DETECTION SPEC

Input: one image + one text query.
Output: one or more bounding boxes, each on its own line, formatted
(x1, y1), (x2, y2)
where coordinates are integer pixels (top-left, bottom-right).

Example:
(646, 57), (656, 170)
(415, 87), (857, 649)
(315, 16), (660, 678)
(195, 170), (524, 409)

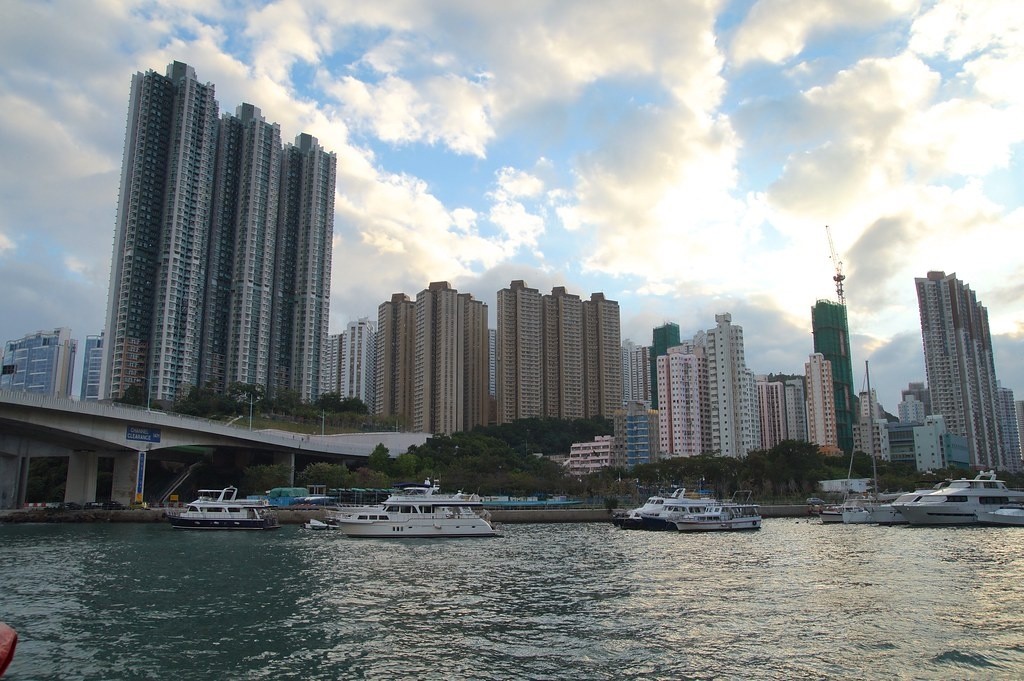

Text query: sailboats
(818, 360), (910, 525)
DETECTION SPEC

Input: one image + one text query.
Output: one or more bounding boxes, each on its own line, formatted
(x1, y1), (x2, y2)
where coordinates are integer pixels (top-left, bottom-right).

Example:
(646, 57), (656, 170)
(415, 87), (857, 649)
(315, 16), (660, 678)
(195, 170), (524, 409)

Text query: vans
(806, 498), (825, 506)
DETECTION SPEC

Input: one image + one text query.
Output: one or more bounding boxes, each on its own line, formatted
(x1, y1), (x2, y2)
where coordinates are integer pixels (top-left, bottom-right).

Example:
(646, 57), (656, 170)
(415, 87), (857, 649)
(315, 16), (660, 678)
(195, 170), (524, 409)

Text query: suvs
(61, 501), (148, 509)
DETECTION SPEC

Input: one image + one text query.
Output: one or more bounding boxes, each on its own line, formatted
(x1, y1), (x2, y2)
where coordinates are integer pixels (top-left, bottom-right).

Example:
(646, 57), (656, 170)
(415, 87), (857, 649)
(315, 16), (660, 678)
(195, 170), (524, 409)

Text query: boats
(674, 501), (761, 530)
(638, 496), (716, 529)
(300, 517), (340, 530)
(841, 491), (910, 523)
(976, 501), (1024, 524)
(163, 485), (281, 530)
(613, 488), (738, 530)
(890, 470), (1024, 526)
(338, 482), (503, 537)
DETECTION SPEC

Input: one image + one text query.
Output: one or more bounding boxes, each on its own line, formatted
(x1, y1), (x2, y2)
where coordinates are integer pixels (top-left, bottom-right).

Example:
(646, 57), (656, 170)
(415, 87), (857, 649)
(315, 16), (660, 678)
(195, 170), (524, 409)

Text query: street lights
(317, 409), (325, 435)
(244, 394), (252, 430)
(142, 376), (151, 412)
